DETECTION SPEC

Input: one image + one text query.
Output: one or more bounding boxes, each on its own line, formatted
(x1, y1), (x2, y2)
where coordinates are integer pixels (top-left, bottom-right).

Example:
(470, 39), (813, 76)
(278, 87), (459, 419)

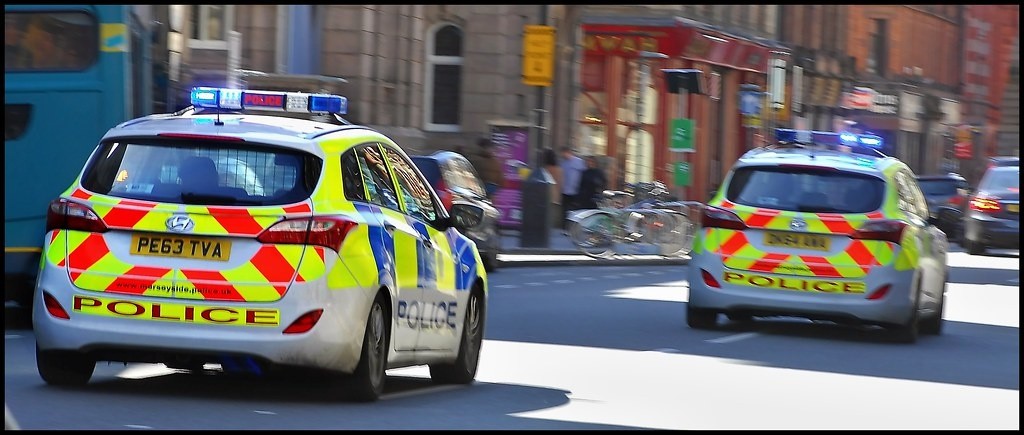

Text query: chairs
(151, 156), (248, 199)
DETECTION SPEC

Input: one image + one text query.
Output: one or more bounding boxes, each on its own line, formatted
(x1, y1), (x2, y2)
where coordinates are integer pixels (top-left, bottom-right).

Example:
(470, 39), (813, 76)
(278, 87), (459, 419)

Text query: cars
(685, 127), (949, 343)
(963, 156), (1019, 249)
(914, 173), (970, 238)
(403, 148), (501, 274)
(31, 88), (487, 404)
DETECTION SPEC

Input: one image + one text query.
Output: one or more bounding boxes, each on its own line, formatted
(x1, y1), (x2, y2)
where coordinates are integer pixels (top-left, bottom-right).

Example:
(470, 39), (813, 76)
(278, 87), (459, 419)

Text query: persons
(469, 137), (608, 249)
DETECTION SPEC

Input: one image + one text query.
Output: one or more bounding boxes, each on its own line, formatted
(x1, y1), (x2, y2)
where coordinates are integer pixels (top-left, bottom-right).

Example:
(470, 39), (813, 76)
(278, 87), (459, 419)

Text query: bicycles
(564, 181), (702, 258)
